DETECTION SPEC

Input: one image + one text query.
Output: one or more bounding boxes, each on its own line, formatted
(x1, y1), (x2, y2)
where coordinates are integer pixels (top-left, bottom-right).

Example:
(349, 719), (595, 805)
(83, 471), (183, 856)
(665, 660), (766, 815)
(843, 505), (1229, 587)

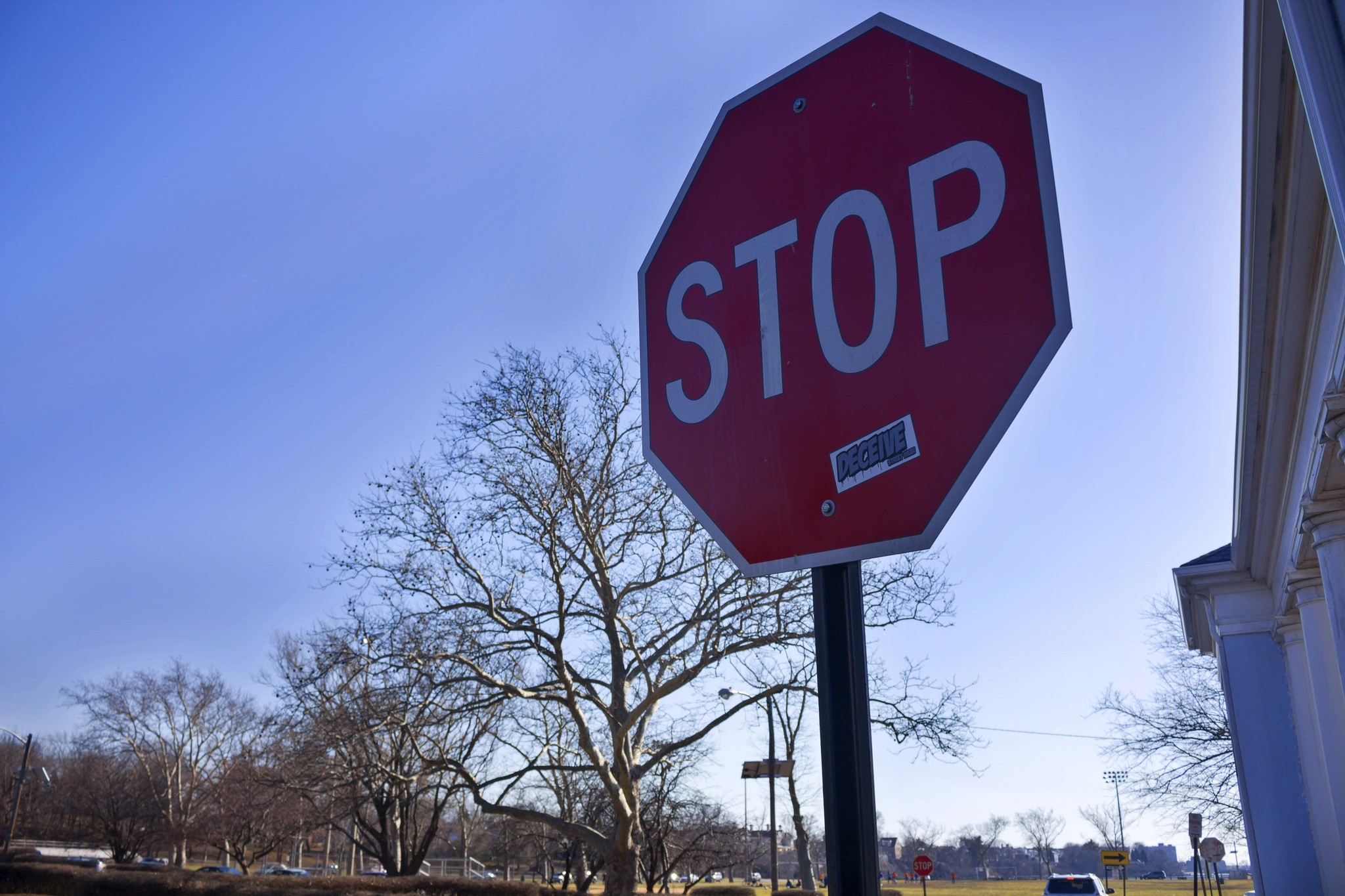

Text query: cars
(141, 855), (763, 884)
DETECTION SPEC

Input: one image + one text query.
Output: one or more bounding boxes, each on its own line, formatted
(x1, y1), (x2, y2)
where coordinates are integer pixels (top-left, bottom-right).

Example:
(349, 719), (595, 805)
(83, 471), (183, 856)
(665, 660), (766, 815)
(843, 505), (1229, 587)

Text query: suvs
(1141, 870), (1167, 880)
(1041, 873), (1115, 896)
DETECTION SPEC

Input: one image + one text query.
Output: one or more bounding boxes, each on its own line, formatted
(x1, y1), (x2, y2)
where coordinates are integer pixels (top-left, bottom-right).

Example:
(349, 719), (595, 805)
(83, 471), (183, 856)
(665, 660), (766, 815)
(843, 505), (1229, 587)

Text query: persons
(785, 867), (957, 891)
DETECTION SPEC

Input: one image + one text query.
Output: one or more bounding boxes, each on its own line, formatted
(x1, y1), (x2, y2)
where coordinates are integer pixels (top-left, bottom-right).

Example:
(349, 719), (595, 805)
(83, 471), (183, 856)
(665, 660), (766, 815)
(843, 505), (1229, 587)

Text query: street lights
(719, 687), (780, 893)
(1103, 771), (1129, 880)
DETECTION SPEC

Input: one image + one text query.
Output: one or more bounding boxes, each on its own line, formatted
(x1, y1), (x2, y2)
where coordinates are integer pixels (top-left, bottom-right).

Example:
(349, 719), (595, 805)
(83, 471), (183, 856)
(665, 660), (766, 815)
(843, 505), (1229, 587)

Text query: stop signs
(1199, 837), (1226, 862)
(911, 854), (935, 878)
(636, 12), (1072, 579)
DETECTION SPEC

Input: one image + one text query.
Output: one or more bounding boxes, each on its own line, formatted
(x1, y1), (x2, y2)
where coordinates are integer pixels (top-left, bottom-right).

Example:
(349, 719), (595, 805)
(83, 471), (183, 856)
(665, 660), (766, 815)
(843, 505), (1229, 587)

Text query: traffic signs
(1188, 813), (1202, 837)
(1105, 865), (1125, 871)
